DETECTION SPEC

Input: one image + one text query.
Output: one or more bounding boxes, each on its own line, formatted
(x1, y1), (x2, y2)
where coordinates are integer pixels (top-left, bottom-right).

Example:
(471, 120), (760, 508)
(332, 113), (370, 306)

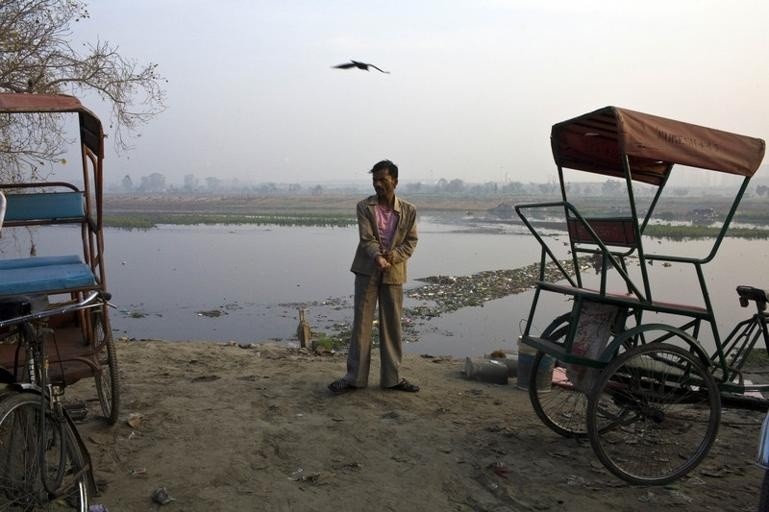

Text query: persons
(328, 160), (420, 392)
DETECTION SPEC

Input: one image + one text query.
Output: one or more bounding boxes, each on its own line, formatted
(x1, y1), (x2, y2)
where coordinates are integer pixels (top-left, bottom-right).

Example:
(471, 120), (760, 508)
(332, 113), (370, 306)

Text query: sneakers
(328, 378), (354, 393)
(382, 378), (420, 392)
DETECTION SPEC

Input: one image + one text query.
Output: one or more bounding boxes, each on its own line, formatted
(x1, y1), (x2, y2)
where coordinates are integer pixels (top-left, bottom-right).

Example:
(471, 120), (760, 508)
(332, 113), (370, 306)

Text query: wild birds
(330, 59), (391, 74)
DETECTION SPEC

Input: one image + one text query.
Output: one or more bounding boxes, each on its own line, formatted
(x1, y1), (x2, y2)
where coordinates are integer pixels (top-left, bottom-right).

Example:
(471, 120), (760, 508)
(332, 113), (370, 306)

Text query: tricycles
(0, 95), (121, 510)
(514, 105), (769, 487)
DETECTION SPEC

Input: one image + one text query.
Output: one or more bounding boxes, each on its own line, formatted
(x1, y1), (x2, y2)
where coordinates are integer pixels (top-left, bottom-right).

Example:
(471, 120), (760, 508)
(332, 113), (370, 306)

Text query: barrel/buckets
(517, 336), (554, 393)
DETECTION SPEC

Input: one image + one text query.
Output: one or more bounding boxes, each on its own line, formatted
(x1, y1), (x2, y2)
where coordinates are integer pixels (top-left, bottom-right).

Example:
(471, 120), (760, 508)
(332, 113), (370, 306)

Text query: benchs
(0, 181), (94, 300)
(537, 212), (711, 327)
